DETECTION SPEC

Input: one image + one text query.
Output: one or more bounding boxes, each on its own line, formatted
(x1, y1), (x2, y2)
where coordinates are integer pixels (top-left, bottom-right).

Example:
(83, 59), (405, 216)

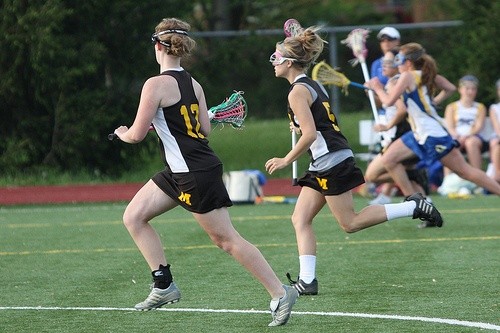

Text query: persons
(114, 18), (297, 326)
(364, 27), (500, 228)
(265, 29), (443, 295)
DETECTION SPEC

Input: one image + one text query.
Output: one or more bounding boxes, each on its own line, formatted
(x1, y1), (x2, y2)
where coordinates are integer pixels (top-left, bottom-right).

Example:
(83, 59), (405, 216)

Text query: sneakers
(286, 272), (319, 295)
(403, 192), (444, 226)
(267, 284), (299, 327)
(135, 281), (181, 312)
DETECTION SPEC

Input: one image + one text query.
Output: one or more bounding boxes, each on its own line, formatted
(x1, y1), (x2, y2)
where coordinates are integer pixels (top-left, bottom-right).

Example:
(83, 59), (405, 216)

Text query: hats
(377, 27), (401, 39)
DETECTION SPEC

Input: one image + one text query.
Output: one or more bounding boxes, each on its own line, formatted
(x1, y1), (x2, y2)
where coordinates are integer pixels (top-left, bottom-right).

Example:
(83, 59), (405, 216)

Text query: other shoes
(366, 194), (393, 205)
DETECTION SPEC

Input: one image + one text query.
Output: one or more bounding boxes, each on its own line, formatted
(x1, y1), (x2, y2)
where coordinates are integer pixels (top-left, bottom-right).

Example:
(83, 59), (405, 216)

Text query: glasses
(394, 54), (408, 65)
(151, 32), (170, 46)
(379, 37), (396, 41)
(269, 52), (303, 66)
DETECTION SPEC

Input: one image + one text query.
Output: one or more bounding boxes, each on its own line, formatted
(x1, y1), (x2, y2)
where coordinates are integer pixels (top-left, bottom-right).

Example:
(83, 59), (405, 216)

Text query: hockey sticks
(311, 61), (374, 90)
(345, 28), (380, 123)
(107, 90), (248, 141)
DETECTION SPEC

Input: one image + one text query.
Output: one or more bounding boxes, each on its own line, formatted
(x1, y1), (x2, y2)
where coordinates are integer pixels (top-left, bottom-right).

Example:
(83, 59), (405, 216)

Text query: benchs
(355, 116), (491, 161)
